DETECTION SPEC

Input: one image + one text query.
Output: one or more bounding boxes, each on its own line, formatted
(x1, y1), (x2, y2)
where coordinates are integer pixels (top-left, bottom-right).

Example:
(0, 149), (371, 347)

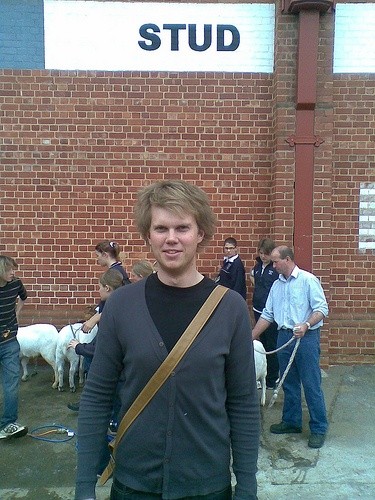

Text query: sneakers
(270, 422), (302, 434)
(0, 422), (28, 441)
(308, 434), (325, 448)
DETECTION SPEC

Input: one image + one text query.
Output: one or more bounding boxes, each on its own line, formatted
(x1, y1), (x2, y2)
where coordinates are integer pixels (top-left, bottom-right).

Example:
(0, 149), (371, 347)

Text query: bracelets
(306, 322), (311, 330)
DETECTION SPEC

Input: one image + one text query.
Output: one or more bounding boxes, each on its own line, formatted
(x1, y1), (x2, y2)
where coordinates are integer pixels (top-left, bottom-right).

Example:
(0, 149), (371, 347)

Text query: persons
(74, 180), (261, 500)
(133, 261), (156, 282)
(216, 237), (246, 301)
(251, 245), (329, 448)
(68, 269), (126, 435)
(251, 239), (279, 389)
(68, 241), (128, 411)
(0, 255), (28, 439)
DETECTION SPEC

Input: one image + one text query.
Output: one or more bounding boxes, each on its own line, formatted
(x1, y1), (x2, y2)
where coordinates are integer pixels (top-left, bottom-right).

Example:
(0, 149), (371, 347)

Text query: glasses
(224, 246), (236, 249)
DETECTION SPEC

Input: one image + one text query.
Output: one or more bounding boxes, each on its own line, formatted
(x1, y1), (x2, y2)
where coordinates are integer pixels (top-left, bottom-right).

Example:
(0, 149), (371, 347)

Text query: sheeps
(16, 324), (58, 389)
(253, 340), (267, 407)
(59, 318), (98, 391)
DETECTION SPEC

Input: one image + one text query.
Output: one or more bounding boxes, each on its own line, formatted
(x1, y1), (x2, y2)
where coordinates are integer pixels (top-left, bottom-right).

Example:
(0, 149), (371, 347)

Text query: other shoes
(275, 378), (279, 383)
(257, 381), (276, 389)
(68, 402), (80, 410)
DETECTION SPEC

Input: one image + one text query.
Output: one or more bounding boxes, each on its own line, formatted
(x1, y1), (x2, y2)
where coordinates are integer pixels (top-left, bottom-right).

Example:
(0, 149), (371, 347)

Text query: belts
(280, 328), (312, 334)
